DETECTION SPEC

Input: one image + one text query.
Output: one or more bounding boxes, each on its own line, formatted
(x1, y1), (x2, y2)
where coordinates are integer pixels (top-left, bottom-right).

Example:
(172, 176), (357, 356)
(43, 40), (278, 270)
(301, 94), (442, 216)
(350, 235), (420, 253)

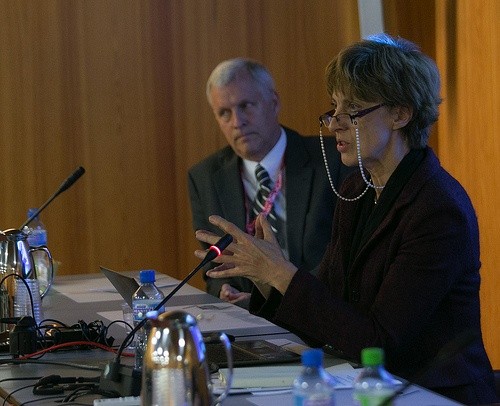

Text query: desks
(0, 270), (464, 406)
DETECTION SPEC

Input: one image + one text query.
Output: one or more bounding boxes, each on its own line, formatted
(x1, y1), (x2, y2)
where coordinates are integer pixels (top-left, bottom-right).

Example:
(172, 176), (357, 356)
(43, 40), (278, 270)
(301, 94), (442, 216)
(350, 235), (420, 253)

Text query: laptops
(98, 265), (301, 368)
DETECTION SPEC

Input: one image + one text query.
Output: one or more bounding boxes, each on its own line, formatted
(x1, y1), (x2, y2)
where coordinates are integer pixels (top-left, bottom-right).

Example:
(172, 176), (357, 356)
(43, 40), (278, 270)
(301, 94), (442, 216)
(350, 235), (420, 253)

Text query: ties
(252, 165), (279, 243)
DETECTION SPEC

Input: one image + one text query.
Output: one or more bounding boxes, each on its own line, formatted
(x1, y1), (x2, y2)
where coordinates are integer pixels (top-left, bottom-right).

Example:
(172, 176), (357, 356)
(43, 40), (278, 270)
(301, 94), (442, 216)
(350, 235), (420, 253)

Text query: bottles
(353, 348), (393, 406)
(23, 208), (48, 293)
(132, 270), (165, 368)
(292, 348), (337, 406)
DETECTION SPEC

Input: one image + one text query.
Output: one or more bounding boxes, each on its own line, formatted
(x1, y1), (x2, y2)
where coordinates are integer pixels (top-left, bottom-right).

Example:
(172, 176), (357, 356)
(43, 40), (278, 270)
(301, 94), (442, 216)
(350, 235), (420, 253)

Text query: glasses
(319, 100), (390, 128)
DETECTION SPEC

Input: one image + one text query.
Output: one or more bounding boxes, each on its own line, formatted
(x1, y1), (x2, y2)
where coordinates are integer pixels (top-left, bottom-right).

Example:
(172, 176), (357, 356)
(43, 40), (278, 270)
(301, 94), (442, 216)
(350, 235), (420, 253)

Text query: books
(218, 366), (306, 387)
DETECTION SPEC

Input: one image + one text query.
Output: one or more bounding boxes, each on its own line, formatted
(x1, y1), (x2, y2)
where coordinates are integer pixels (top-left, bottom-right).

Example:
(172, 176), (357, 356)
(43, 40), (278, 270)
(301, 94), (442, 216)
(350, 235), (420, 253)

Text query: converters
(50, 327), (101, 351)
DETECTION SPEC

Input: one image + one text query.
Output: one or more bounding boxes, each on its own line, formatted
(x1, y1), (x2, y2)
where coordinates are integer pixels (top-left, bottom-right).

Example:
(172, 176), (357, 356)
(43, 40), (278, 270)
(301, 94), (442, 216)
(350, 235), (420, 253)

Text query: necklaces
(373, 196), (377, 205)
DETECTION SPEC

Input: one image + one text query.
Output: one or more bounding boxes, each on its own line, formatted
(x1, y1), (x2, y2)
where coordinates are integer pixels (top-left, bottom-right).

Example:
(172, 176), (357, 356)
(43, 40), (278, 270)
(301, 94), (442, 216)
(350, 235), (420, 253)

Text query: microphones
(97, 233), (233, 396)
(18, 166), (85, 231)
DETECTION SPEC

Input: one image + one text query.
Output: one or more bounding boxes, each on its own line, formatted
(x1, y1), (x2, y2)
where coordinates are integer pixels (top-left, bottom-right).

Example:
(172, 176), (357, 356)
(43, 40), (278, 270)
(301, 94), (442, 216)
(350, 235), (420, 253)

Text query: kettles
(0, 228), (53, 343)
(141, 311), (235, 406)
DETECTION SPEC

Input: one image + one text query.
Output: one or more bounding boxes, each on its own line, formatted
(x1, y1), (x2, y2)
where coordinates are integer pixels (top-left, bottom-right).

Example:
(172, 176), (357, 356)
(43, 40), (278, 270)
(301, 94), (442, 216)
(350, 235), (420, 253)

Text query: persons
(187, 57), (357, 311)
(194, 35), (500, 406)
